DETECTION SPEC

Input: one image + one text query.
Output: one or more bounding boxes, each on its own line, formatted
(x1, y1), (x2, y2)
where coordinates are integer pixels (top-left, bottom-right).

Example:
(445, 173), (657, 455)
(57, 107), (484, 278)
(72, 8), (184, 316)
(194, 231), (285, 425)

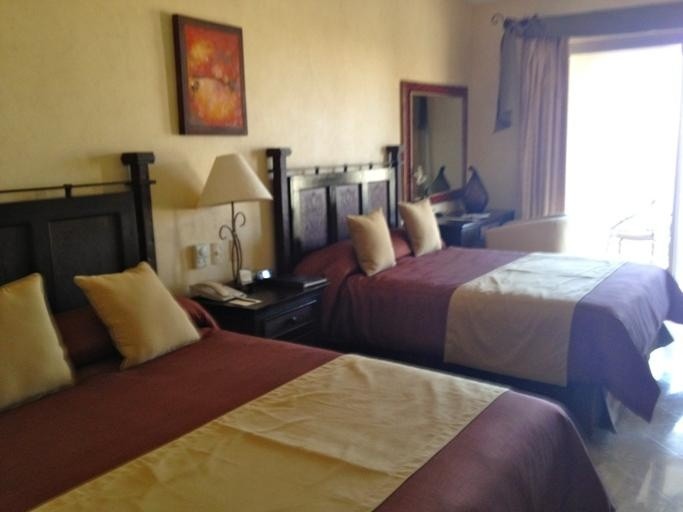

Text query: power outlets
(191, 243), (209, 269)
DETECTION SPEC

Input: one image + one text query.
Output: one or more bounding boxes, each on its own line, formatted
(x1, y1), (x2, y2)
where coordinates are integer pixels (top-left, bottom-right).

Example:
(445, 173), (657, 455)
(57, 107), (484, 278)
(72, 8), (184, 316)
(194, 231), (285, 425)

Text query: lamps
(191, 151), (273, 289)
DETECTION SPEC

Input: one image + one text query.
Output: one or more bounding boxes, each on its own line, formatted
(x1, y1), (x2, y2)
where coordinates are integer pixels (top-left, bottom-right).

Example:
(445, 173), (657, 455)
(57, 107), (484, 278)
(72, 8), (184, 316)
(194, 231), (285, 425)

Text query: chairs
(484, 210), (568, 253)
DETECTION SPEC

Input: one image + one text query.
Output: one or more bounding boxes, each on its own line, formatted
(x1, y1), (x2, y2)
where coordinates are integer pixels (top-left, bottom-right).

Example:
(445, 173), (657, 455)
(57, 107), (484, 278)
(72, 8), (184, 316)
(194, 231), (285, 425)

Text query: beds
(265, 145), (680, 434)
(0, 148), (615, 511)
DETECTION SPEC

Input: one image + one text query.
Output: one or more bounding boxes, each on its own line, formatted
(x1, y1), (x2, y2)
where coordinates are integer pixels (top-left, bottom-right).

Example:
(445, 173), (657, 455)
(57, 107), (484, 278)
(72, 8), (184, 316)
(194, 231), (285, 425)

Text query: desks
(434, 203), (513, 248)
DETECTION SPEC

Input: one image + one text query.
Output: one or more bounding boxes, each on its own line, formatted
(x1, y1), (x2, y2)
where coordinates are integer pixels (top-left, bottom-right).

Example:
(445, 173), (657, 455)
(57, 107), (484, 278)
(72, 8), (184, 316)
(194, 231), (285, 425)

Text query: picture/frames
(170, 9), (248, 136)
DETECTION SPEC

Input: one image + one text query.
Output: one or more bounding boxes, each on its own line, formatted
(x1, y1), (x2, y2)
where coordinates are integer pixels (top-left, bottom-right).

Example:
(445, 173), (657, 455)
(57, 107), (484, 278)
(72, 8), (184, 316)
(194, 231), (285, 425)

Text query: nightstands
(188, 272), (328, 348)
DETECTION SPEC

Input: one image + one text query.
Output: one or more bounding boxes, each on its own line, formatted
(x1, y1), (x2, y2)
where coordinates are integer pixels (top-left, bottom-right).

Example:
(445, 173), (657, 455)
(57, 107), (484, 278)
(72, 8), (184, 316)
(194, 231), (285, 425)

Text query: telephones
(189, 280), (244, 302)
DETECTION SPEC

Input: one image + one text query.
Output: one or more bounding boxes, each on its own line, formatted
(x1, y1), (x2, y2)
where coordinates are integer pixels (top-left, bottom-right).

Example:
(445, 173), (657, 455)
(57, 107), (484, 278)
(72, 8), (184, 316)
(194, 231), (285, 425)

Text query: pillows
(66, 252), (203, 374)
(338, 201), (398, 279)
(0, 273), (72, 415)
(398, 194), (445, 257)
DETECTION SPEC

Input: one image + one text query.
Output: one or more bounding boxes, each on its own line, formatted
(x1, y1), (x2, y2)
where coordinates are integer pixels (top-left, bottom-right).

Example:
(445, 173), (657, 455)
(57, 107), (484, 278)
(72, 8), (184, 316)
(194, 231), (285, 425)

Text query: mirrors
(399, 78), (467, 211)
